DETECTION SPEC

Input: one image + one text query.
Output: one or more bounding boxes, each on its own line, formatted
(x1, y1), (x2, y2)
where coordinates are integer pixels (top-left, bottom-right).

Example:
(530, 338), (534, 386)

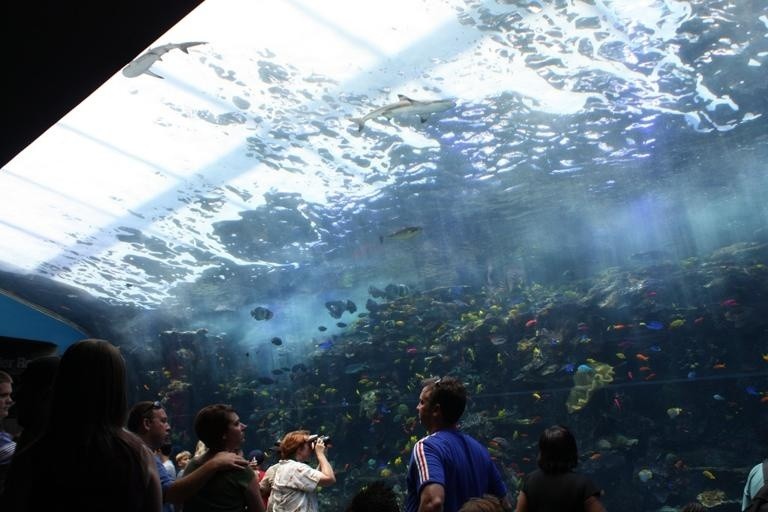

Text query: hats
(304, 434), (319, 443)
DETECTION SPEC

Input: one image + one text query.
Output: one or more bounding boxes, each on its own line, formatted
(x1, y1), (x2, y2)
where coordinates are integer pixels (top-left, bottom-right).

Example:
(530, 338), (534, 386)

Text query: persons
(0, 340), (162, 511)
(512, 421), (605, 512)
(404, 373), (508, 512)
(739, 458), (768, 512)
(125, 402), (336, 511)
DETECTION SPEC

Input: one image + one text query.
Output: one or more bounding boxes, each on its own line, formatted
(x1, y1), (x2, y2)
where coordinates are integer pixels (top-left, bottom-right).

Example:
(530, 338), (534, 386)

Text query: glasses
(144, 400), (163, 414)
(434, 377), (441, 389)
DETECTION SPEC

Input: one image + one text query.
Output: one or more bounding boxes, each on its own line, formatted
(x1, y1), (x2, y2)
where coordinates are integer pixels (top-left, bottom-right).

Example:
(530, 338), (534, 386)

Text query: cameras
(309, 435), (330, 451)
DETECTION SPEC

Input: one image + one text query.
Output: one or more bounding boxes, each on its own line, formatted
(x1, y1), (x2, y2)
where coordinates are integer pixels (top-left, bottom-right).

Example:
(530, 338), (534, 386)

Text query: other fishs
(135, 284), (768, 510)
